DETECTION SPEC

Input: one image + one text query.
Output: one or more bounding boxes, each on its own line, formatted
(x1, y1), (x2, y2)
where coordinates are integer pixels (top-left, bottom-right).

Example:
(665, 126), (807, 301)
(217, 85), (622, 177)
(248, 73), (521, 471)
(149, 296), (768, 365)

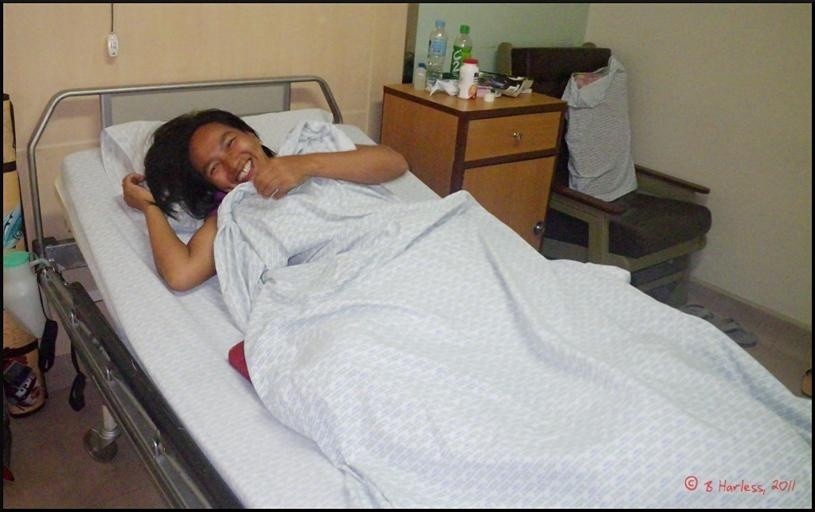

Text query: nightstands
(379, 81), (568, 255)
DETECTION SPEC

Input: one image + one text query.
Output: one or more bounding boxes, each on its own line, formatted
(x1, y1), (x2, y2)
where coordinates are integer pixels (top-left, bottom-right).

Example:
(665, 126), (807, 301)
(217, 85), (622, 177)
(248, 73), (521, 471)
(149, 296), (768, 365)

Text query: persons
(120, 108), (411, 292)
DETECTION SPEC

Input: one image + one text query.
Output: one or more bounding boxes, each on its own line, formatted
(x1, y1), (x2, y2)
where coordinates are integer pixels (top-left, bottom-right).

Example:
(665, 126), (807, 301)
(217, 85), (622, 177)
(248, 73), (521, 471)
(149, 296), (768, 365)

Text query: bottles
(413, 19), (477, 100)
(3, 251), (49, 341)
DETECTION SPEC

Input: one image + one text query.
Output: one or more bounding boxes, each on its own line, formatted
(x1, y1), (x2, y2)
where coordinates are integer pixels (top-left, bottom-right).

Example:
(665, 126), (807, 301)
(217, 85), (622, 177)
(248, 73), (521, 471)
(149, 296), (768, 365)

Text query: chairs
(494, 40), (712, 308)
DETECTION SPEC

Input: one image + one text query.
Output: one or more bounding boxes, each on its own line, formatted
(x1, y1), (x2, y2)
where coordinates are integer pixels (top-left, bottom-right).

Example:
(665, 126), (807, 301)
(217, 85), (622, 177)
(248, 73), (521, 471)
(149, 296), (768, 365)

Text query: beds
(59, 106), (813, 508)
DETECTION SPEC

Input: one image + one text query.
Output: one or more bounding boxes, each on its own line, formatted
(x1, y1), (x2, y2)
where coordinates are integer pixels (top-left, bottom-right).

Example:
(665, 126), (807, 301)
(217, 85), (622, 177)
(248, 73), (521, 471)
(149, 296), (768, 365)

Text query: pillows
(100, 108), (339, 236)
(561, 53), (640, 203)
